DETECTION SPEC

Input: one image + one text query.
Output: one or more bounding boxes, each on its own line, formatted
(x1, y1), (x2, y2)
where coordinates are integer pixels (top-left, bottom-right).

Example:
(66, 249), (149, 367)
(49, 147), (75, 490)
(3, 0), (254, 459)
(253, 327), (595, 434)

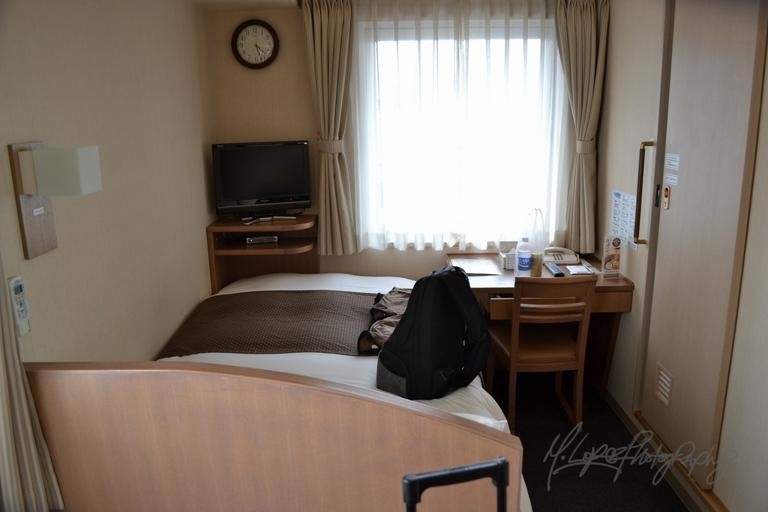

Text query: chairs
(486, 274), (598, 434)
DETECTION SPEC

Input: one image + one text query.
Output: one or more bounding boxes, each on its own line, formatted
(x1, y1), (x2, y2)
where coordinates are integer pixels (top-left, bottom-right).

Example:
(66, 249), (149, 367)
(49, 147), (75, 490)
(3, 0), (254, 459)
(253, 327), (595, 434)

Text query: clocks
(231, 19), (280, 70)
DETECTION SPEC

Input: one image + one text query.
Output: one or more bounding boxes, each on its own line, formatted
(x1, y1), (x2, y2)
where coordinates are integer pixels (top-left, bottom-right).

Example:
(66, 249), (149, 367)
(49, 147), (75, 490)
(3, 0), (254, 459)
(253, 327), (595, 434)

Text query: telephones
(542, 247), (580, 264)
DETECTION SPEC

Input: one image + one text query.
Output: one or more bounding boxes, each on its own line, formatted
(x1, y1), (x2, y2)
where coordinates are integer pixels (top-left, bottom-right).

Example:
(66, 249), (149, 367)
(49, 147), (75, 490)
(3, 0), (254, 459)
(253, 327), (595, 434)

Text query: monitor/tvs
(211, 140), (311, 218)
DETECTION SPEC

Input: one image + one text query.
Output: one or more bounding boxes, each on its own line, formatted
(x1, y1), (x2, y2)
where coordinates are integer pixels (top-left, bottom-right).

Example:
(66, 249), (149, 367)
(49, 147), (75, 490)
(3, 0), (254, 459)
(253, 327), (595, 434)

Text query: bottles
(515, 237), (532, 278)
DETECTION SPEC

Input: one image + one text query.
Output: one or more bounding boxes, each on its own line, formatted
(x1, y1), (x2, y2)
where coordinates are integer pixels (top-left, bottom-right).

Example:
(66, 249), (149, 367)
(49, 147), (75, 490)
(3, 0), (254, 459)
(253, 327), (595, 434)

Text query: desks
(446, 253), (635, 398)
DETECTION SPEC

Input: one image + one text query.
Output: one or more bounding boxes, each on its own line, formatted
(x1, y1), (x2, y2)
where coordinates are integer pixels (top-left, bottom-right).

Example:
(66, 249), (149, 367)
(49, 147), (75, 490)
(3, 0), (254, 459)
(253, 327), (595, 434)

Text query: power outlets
(7, 274), (32, 339)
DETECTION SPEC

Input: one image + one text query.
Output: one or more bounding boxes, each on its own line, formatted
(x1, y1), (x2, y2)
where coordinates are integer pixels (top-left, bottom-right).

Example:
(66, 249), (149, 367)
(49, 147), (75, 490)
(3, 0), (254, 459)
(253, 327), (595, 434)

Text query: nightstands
(205, 215), (319, 294)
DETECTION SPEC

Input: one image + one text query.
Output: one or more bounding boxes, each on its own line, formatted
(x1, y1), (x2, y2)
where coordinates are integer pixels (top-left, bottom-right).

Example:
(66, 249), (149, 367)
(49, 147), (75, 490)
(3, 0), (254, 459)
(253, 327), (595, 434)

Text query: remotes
(544, 261), (565, 278)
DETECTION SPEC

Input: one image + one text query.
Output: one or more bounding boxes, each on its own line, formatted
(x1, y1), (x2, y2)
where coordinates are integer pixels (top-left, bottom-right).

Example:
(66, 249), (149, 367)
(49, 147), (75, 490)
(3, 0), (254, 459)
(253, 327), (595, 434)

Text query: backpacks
(376, 266), (507, 400)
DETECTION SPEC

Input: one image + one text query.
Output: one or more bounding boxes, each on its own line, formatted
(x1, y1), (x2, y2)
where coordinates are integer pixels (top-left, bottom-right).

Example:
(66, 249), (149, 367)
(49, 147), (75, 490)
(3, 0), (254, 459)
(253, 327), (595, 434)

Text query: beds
(23, 274), (524, 512)
